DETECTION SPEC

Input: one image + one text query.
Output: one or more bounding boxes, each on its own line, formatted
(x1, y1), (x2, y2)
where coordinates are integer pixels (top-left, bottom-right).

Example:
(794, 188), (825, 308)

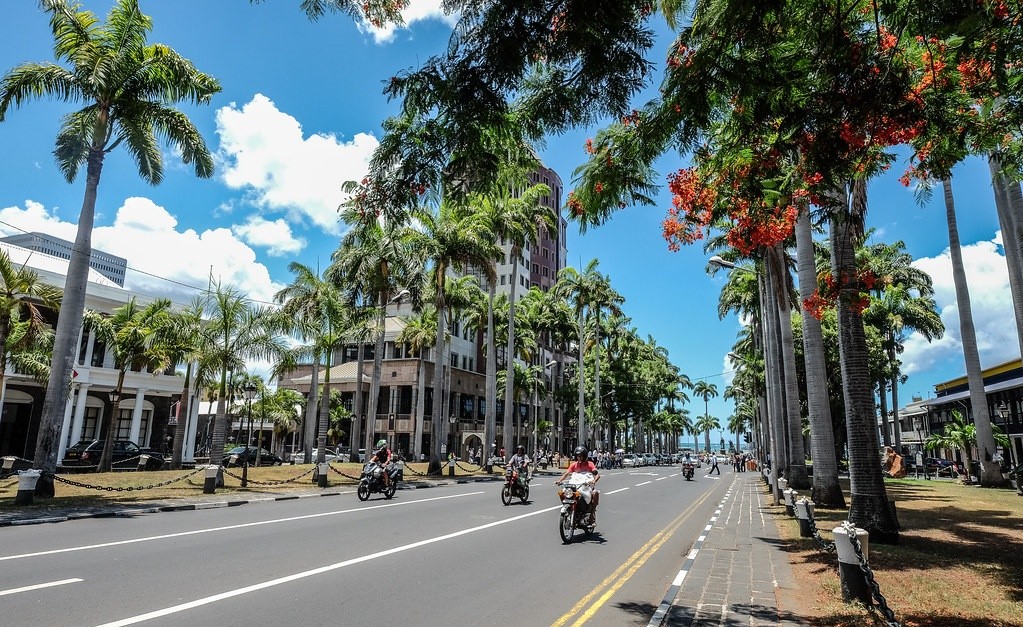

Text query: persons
(370, 439), (390, 488)
(532, 448), (624, 469)
(447, 446), (504, 462)
(506, 444), (529, 497)
(667, 449), (770, 477)
(555, 446), (600, 529)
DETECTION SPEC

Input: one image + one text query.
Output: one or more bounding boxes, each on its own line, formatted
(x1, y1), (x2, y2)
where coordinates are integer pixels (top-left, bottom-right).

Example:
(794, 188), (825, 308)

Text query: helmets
(574, 446), (588, 463)
(516, 445), (525, 457)
(376, 439), (388, 452)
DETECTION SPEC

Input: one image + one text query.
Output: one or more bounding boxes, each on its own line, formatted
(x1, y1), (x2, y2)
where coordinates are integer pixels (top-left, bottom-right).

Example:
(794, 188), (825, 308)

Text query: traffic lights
(743, 432), (751, 444)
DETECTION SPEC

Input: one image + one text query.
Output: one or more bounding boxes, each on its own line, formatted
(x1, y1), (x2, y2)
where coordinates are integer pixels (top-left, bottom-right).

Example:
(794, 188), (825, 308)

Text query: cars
(61, 440), (164, 469)
(620, 452), (683, 469)
(337, 448), (366, 463)
(923, 458), (957, 478)
(684, 452), (730, 469)
(222, 446), (283, 469)
(294, 448), (340, 464)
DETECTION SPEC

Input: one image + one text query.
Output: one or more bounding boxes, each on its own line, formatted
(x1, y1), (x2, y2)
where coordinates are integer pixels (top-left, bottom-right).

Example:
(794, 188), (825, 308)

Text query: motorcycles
(555, 481), (597, 543)
(356, 462), (399, 502)
(501, 460), (534, 506)
(682, 461), (695, 481)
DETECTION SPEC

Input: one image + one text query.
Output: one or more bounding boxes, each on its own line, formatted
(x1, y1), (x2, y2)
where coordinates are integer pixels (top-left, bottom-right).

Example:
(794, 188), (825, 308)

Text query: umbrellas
(615, 448), (624, 453)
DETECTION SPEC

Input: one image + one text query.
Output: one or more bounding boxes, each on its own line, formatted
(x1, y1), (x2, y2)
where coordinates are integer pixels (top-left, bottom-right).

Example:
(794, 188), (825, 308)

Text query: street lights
(709, 254), (780, 506)
(534, 360), (557, 471)
(914, 420), (932, 480)
(349, 414), (357, 453)
(727, 352), (764, 475)
(240, 381), (256, 487)
(448, 412), (458, 477)
